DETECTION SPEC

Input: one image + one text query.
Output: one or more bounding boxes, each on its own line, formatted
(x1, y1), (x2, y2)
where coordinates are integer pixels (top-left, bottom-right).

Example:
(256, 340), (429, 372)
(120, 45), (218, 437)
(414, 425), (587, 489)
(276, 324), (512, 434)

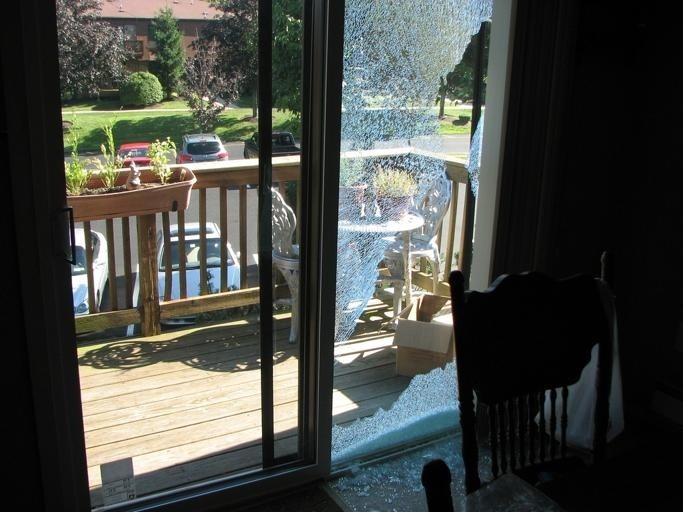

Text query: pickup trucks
(244, 130), (302, 159)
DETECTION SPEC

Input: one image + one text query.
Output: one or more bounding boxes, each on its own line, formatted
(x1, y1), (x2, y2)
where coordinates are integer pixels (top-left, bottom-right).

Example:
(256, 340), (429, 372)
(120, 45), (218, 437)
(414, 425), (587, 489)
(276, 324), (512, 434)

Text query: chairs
(382, 170), (451, 324)
(450, 250), (649, 512)
(272, 187), (300, 344)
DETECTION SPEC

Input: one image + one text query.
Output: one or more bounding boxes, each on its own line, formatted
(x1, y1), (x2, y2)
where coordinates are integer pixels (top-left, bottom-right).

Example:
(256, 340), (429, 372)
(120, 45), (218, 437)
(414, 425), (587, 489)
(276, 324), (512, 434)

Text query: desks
(339, 209), (425, 331)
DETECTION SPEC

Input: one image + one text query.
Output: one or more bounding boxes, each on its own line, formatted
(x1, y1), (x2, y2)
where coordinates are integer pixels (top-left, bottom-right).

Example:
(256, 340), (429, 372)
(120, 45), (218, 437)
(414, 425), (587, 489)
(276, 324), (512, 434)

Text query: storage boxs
(380, 293), (455, 377)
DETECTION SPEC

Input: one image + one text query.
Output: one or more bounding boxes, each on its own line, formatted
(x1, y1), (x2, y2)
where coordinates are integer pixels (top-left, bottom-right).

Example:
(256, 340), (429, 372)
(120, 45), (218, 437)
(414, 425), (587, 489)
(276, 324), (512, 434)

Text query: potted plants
(66, 106), (197, 221)
(338, 154), (417, 222)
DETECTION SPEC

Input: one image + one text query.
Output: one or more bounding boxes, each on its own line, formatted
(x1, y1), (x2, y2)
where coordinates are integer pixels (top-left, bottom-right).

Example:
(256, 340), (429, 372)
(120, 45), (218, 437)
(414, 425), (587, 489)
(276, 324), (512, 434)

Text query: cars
(69, 221), (240, 326)
(114, 134), (229, 168)
